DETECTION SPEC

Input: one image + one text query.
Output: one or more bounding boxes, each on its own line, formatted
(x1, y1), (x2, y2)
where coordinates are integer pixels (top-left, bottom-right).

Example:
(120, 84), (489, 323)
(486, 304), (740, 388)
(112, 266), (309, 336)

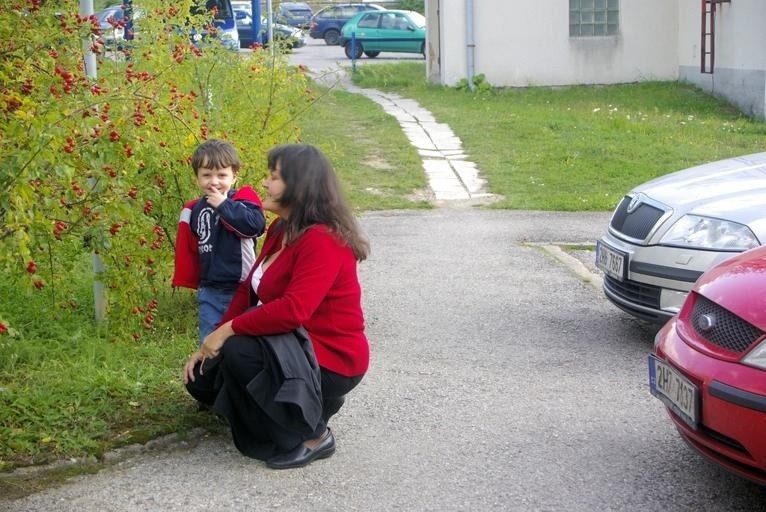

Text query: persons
(173, 138), (268, 344)
(182, 144), (370, 469)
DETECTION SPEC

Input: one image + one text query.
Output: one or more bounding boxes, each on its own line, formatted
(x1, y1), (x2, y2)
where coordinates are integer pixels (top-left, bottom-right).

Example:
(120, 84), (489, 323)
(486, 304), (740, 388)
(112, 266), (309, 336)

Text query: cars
(587, 150), (766, 323)
(644, 242), (766, 492)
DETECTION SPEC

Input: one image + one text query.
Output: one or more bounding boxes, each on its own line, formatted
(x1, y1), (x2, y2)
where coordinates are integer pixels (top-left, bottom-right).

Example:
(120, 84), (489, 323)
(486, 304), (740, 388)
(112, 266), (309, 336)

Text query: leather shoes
(266, 426), (334, 468)
